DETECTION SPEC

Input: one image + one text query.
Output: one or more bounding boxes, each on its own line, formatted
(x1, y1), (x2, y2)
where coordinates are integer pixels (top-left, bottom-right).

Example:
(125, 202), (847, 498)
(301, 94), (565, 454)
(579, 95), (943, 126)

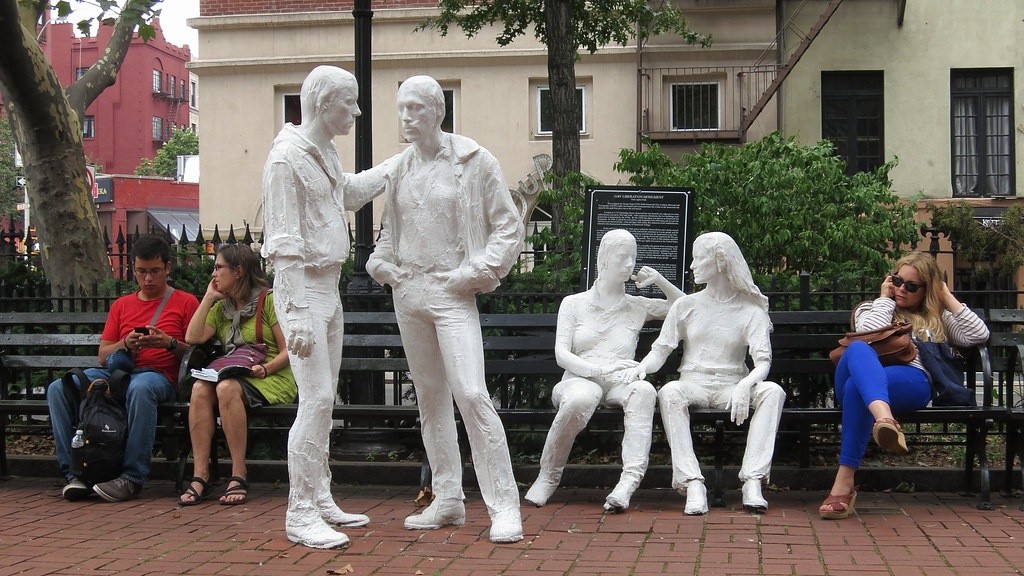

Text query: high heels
(872, 418), (908, 457)
(820, 489), (857, 519)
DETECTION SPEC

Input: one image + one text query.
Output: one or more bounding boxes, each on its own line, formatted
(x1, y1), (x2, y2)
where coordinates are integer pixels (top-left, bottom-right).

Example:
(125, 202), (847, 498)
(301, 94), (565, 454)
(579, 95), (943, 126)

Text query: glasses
(892, 276), (925, 293)
(213, 265), (231, 271)
(134, 265), (166, 277)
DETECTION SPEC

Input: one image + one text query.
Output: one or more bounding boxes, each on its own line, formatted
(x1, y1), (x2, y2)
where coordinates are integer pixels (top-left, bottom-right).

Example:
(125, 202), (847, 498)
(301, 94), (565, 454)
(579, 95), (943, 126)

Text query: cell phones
(134, 326), (150, 336)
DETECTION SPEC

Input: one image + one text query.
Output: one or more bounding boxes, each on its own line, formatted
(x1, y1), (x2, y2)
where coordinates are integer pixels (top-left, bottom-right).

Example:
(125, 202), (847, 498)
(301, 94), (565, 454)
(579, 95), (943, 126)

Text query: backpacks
(62, 368), (131, 483)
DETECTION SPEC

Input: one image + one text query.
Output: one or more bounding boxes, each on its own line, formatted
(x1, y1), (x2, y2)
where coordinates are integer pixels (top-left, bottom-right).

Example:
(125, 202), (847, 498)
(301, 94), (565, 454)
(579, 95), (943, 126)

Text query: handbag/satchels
(107, 349), (135, 374)
(918, 342), (975, 405)
(206, 342), (268, 377)
(829, 301), (916, 366)
(177, 346), (210, 402)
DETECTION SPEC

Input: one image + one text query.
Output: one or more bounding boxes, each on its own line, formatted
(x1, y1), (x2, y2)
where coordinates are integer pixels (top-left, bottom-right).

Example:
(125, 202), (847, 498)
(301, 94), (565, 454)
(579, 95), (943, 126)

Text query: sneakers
(63, 478), (95, 502)
(92, 478), (130, 502)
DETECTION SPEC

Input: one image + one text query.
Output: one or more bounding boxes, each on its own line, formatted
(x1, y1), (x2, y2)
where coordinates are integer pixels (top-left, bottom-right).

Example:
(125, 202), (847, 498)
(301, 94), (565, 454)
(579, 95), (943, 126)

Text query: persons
(818, 250), (989, 518)
(257, 65), (407, 553)
(180, 244), (297, 505)
(618, 230), (786, 515)
(367, 74), (525, 545)
(525, 228), (688, 511)
(47, 235), (200, 503)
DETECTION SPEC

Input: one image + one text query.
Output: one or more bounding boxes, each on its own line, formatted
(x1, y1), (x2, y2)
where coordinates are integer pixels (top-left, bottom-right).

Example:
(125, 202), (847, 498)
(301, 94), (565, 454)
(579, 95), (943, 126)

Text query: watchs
(165, 336), (178, 351)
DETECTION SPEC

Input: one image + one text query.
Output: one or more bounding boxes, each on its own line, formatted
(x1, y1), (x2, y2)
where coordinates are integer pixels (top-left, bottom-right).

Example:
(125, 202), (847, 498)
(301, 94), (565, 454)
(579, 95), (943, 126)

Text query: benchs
(0, 309), (1024, 509)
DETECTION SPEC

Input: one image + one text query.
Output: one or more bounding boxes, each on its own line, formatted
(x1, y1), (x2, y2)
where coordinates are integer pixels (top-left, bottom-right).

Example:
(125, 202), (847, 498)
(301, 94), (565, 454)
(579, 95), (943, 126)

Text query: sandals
(220, 476), (250, 504)
(178, 477), (215, 506)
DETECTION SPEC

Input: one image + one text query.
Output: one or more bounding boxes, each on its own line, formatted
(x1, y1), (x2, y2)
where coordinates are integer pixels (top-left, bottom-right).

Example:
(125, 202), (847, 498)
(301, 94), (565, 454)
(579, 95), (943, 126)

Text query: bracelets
(123, 337), (129, 349)
(262, 364), (267, 378)
